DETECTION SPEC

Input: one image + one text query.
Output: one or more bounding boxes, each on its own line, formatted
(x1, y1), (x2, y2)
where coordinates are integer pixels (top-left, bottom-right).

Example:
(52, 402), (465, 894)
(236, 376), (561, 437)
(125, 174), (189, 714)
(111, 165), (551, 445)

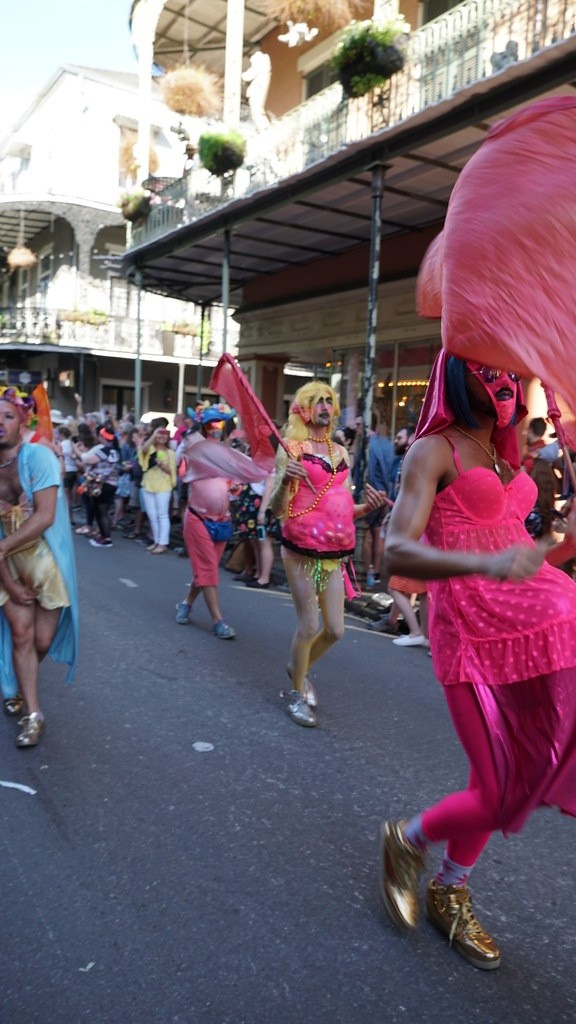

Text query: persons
(379, 349), (576, 968)
(183, 143), (198, 176)
(519, 417), (576, 545)
(242, 52), (272, 130)
(50, 394), (429, 645)
(0, 381), (79, 745)
(268, 382), (387, 726)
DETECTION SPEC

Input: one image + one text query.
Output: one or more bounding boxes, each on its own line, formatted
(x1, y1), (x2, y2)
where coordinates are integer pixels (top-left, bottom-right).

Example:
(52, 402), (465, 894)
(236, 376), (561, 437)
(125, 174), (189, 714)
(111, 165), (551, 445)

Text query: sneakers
(74, 526), (89, 533)
(286, 662), (318, 707)
(366, 617), (400, 633)
(401, 634), (430, 648)
(212, 620), (237, 638)
(16, 710), (44, 746)
(176, 603), (191, 623)
(89, 537), (112, 547)
(393, 635), (425, 646)
(4, 693), (24, 715)
(426, 879), (500, 969)
(281, 690), (316, 726)
(379, 820), (431, 932)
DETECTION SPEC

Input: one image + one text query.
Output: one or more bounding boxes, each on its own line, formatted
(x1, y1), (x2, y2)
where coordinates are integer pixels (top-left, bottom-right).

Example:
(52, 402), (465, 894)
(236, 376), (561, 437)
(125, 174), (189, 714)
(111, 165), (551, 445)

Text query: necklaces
(0, 459), (13, 468)
(454, 425), (500, 474)
(288, 433), (336, 517)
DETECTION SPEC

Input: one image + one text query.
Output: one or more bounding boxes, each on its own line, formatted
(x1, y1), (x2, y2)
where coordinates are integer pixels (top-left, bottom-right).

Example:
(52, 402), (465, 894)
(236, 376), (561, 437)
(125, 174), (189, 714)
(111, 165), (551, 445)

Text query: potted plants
(119, 190), (154, 221)
(327, 11), (410, 96)
(195, 127), (245, 175)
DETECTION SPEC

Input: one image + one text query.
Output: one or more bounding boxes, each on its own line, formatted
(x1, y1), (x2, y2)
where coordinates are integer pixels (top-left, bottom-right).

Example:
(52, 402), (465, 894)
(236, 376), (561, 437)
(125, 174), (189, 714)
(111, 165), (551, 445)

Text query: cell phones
(161, 430), (170, 435)
(72, 436), (78, 444)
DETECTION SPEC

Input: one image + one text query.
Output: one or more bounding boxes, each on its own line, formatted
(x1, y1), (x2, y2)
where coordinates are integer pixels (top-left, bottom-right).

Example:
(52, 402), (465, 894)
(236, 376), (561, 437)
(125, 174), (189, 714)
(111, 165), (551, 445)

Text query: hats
(200, 408), (226, 423)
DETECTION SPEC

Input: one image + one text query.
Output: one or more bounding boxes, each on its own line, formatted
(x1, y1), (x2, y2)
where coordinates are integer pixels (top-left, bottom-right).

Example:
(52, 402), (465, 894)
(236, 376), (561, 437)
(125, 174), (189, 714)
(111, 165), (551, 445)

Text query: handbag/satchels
(188, 506), (234, 541)
(75, 470), (105, 497)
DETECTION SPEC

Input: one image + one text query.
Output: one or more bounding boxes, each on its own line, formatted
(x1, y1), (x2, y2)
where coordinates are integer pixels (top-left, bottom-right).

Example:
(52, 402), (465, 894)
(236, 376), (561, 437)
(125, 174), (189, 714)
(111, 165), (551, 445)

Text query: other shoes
(176, 546), (269, 588)
(152, 546), (167, 553)
(145, 544), (157, 551)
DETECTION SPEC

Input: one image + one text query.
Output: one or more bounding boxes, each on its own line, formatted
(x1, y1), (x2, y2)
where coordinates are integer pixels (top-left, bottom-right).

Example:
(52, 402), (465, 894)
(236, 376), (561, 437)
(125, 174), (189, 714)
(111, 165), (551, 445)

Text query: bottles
(366, 564), (375, 590)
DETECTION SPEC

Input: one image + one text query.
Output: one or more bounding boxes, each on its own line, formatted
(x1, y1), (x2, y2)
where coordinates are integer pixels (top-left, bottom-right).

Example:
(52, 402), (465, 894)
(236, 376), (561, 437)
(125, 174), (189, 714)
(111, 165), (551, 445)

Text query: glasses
(464, 366), (522, 383)
